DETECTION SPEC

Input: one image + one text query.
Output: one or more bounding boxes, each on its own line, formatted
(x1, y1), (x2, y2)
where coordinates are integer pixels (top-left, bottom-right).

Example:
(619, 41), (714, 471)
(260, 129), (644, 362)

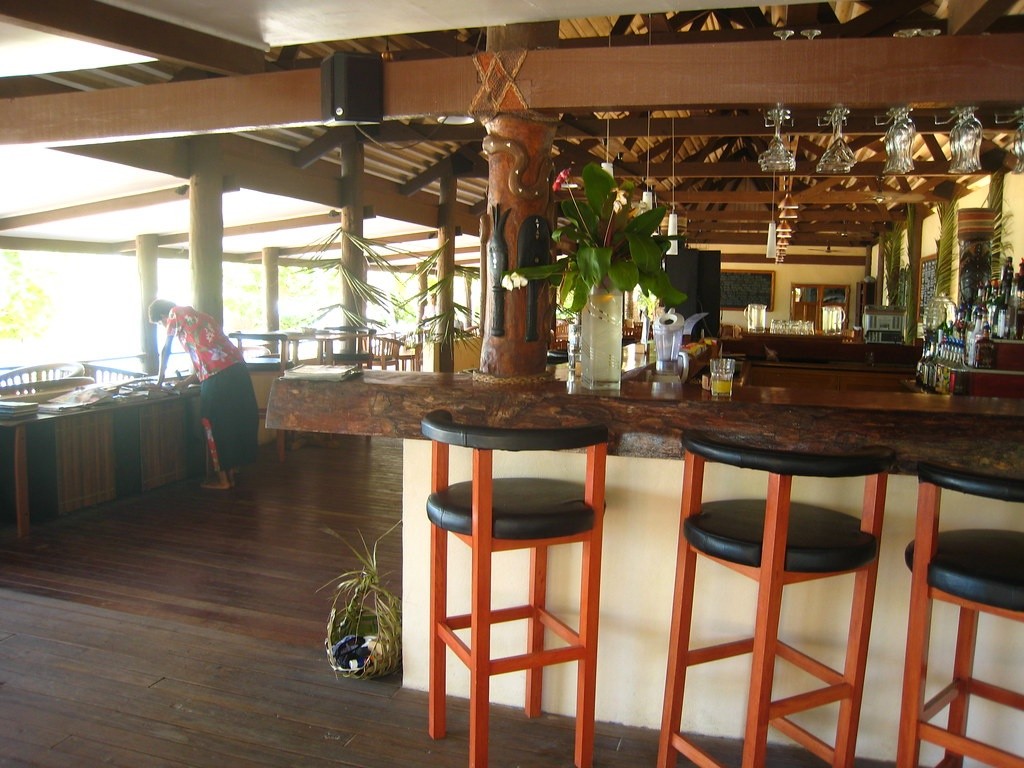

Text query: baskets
(315, 520), (402, 682)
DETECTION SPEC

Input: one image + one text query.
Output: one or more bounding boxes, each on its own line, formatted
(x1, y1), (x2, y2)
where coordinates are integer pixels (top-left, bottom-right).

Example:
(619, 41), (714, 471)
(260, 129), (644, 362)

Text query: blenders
(649, 308), (689, 383)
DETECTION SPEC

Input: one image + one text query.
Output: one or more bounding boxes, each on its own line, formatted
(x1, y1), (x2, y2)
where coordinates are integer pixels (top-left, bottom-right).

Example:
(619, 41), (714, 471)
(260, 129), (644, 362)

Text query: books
(283, 364), (360, 381)
(0, 376), (195, 420)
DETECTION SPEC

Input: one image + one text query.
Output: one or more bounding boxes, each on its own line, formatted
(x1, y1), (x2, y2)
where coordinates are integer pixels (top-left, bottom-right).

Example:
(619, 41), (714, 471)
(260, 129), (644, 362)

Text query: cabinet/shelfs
(961, 339), (1024, 397)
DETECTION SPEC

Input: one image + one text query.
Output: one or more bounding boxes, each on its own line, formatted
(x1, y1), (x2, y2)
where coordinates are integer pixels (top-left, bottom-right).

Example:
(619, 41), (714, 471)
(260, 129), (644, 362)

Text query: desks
(0, 386), (200, 539)
(254, 326), (365, 444)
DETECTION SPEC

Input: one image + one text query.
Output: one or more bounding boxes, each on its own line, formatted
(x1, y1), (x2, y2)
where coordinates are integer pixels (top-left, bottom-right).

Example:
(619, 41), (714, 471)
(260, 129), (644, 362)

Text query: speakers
(321, 51), (383, 123)
(663, 249), (721, 339)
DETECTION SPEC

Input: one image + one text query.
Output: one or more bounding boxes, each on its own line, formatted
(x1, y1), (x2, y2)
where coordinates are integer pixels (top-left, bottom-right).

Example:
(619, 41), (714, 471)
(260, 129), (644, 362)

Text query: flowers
(500, 161), (687, 318)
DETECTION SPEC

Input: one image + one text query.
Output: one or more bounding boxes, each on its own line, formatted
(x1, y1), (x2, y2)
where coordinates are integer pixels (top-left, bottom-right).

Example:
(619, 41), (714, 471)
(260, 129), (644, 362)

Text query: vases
(579, 291), (623, 392)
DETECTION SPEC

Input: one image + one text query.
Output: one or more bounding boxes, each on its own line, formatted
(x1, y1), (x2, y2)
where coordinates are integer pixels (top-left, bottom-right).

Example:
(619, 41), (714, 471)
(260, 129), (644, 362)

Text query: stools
(657, 426), (898, 768)
(896, 461), (1024, 768)
(229, 331), (294, 461)
(422, 409), (608, 768)
(324, 326), (376, 444)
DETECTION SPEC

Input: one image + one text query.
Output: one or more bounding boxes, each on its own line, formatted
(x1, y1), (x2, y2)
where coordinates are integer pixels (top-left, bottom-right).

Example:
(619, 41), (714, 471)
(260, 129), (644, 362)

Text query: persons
(148, 299), (258, 489)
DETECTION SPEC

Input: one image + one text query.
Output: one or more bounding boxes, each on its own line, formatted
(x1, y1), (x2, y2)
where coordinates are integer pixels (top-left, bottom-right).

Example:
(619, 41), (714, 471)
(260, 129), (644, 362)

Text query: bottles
(916, 258), (1024, 394)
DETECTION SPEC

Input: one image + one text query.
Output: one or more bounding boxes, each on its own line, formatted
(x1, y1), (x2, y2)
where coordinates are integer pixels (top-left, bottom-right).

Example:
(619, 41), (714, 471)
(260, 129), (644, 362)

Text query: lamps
(766, 172), (776, 258)
(665, 112), (678, 255)
(601, 112), (613, 177)
(642, 110), (652, 209)
(776, 210), (798, 262)
(778, 169), (798, 209)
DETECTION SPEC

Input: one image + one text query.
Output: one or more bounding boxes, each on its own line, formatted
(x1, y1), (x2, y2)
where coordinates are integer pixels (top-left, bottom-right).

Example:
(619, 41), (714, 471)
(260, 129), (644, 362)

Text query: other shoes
(200, 477), (235, 490)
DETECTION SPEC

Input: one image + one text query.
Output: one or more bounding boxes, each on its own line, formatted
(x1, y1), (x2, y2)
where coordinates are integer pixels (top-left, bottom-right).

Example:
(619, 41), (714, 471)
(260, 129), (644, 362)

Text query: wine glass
(947, 106), (983, 174)
(883, 107), (917, 176)
(761, 113), (796, 171)
(816, 108), (858, 175)
(758, 108), (793, 163)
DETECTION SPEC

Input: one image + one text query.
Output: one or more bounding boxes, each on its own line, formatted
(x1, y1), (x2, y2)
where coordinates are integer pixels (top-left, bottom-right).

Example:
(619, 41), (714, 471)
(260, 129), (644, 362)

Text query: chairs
(0, 378), (95, 403)
(0, 362), (86, 386)
(547, 318), (656, 350)
(368, 327), (482, 372)
(82, 360), (150, 391)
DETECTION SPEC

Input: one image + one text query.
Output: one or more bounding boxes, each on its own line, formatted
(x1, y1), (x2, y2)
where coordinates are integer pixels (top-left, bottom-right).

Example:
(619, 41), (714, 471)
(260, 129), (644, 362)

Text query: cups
(710, 359), (734, 396)
(770, 320), (814, 335)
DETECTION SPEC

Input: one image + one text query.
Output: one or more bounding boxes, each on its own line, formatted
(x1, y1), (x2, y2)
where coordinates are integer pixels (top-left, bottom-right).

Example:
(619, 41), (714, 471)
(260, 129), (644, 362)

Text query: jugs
(822, 306), (845, 335)
(744, 304), (767, 333)
